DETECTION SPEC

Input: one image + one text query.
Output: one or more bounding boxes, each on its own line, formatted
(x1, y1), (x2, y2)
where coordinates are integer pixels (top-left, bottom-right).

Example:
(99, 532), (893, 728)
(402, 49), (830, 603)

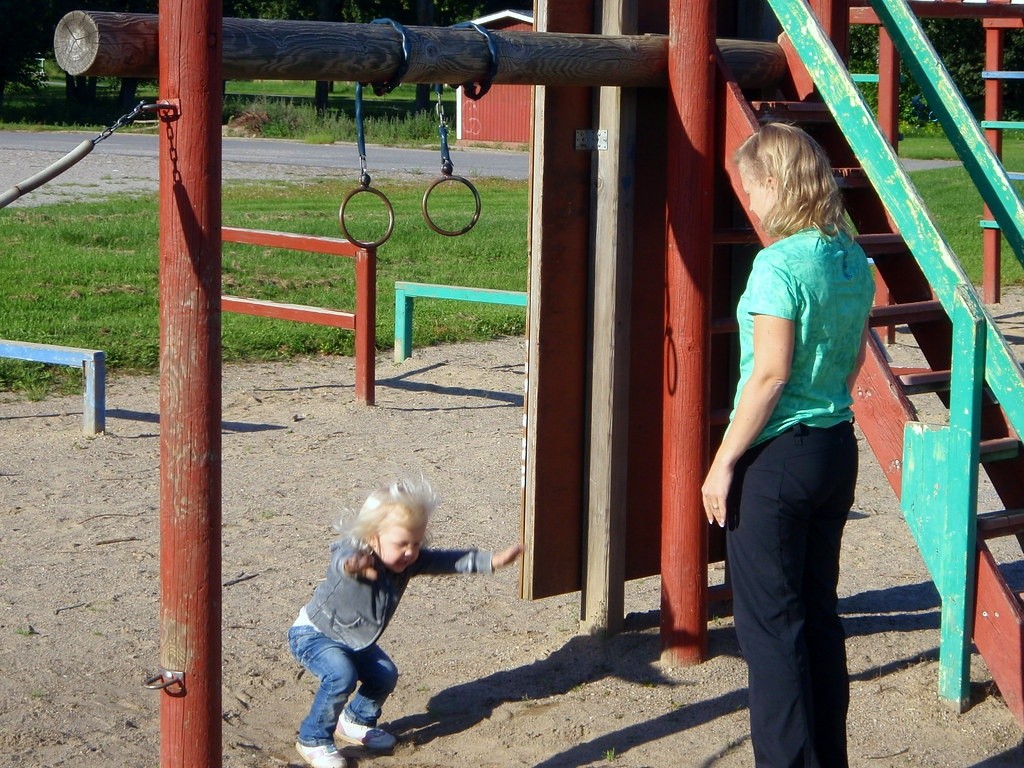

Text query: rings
(712, 506), (719, 510)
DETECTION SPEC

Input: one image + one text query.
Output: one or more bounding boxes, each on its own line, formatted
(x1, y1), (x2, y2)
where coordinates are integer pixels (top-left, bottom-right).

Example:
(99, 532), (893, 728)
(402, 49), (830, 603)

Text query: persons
(290, 485), (524, 768)
(702, 122), (877, 768)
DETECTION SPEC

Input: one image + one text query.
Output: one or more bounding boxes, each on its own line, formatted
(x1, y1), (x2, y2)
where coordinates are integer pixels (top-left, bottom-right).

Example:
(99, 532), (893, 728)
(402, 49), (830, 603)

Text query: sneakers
(296, 742), (347, 768)
(335, 709), (395, 750)
